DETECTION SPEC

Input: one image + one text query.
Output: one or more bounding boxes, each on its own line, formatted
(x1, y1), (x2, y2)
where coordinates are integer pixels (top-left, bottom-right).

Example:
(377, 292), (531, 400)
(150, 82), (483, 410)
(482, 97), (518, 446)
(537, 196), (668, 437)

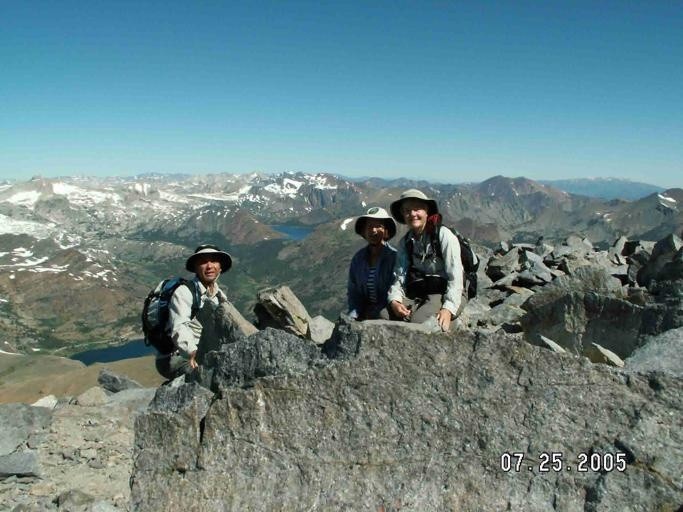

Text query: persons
(380, 189), (469, 332)
(347, 207), (398, 322)
(156, 244), (233, 379)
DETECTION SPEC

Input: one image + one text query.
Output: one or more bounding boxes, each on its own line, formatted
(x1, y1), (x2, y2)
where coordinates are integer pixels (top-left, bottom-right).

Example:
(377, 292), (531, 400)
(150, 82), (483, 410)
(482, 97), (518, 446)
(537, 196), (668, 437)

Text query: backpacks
(141, 276), (221, 356)
(404, 225), (480, 300)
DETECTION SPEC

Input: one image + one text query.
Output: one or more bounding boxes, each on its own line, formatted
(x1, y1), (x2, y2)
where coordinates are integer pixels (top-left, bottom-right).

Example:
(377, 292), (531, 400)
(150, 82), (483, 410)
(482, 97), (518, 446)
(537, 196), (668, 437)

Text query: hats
(390, 189), (438, 224)
(185, 244), (232, 274)
(355, 207), (396, 241)
